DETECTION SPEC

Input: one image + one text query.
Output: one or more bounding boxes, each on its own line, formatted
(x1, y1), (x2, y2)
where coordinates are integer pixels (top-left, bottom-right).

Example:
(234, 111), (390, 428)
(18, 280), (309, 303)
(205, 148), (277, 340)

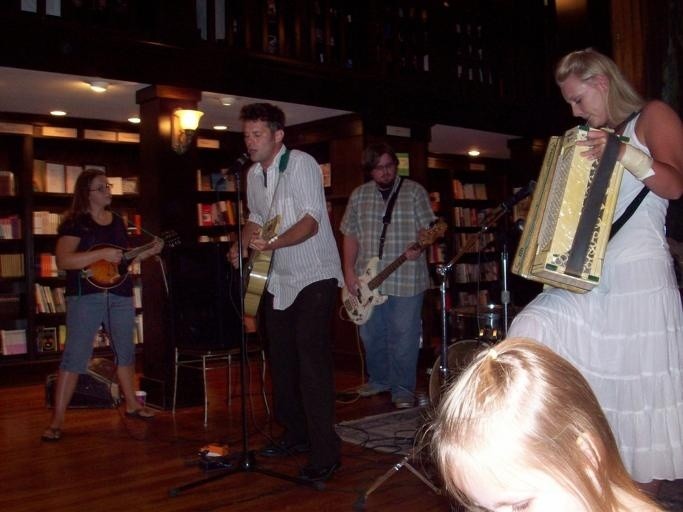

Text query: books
(93, 325), (109, 348)
(429, 276), (450, 290)
(0, 214), (22, 240)
(199, 231), (238, 243)
(427, 156), (450, 169)
(197, 166), (237, 191)
(454, 206), (497, 228)
(132, 284), (143, 309)
(395, 152), (410, 176)
(32, 211), (65, 234)
(33, 159), (140, 194)
(38, 324), (67, 353)
(34, 282), (66, 314)
(454, 261), (501, 283)
(197, 200), (238, 227)
(0, 294), (23, 319)
(0, 171), (21, 195)
(429, 192), (442, 213)
(319, 161), (331, 188)
(128, 261), (140, 275)
(0, 254), (25, 278)
(426, 241), (446, 263)
(0, 329), (28, 355)
(458, 289), (489, 307)
(469, 162), (486, 171)
(39, 251), (66, 277)
(453, 179), (486, 200)
(121, 212), (142, 234)
(454, 232), (495, 252)
(133, 313), (144, 344)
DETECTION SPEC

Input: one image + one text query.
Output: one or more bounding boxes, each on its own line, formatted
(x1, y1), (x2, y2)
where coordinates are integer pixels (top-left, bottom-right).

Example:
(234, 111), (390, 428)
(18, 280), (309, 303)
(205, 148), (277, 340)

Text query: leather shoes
(356, 383), (390, 396)
(393, 397), (415, 409)
(262, 437), (297, 454)
(295, 459), (340, 481)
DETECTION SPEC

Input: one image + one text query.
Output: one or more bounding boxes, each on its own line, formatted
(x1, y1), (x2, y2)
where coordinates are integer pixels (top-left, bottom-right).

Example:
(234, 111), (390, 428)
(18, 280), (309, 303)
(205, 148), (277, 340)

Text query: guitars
(82, 228), (183, 289)
(342, 221), (449, 325)
(243, 215), (281, 316)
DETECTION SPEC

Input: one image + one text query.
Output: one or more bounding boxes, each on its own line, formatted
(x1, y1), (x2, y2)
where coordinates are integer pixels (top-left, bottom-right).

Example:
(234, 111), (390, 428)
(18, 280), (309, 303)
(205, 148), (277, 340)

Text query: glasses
(89, 183), (114, 192)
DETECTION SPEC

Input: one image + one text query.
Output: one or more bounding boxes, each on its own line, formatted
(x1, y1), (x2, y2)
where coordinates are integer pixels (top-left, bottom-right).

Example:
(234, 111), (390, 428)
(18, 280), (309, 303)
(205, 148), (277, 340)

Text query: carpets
(333, 404), (436, 461)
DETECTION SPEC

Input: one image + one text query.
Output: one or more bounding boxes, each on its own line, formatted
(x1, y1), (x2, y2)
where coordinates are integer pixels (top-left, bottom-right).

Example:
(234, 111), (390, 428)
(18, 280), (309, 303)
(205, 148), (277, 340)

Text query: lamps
(90, 82), (109, 93)
(220, 98), (236, 106)
(171, 106), (204, 154)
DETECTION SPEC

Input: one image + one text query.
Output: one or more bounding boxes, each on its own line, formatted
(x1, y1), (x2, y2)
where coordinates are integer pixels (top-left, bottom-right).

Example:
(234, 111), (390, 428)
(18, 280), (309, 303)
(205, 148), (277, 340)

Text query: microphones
(486, 219), (525, 249)
(504, 179), (539, 210)
(216, 154), (251, 187)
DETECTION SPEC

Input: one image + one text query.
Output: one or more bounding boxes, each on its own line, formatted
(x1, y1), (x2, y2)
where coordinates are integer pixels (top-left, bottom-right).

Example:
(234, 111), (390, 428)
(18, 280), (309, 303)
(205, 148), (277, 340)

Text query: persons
(429, 336), (666, 512)
(228, 102), (346, 480)
(340, 144), (435, 409)
(40, 168), (156, 442)
(504, 47), (683, 495)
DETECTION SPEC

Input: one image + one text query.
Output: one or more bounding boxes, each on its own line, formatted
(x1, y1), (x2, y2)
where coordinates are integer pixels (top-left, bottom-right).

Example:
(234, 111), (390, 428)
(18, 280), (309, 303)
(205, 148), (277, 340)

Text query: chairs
(152, 243), (270, 424)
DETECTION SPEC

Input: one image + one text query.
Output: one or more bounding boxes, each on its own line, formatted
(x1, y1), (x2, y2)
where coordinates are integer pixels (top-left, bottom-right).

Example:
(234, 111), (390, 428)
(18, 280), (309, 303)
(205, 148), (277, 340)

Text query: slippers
(41, 426), (62, 441)
(124, 407), (155, 420)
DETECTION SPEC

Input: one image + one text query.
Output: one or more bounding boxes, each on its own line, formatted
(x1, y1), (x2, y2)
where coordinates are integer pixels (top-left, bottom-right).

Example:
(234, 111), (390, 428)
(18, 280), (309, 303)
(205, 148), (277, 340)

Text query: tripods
(170, 173), (327, 499)
(359, 438), (443, 502)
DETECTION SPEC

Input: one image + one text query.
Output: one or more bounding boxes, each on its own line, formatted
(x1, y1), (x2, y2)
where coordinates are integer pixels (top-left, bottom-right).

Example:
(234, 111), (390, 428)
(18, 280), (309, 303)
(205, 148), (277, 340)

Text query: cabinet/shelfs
(282, 114), (364, 356)
(195, 128), (256, 342)
(30, 134), (144, 370)
(450, 168), (502, 307)
(423, 176), (454, 337)
(0, 133), (30, 378)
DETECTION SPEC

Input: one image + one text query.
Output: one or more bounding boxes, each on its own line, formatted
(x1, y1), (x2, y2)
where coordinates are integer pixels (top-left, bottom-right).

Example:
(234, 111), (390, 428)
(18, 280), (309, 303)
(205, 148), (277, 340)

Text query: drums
(429, 339), (494, 408)
(448, 306), (523, 344)
